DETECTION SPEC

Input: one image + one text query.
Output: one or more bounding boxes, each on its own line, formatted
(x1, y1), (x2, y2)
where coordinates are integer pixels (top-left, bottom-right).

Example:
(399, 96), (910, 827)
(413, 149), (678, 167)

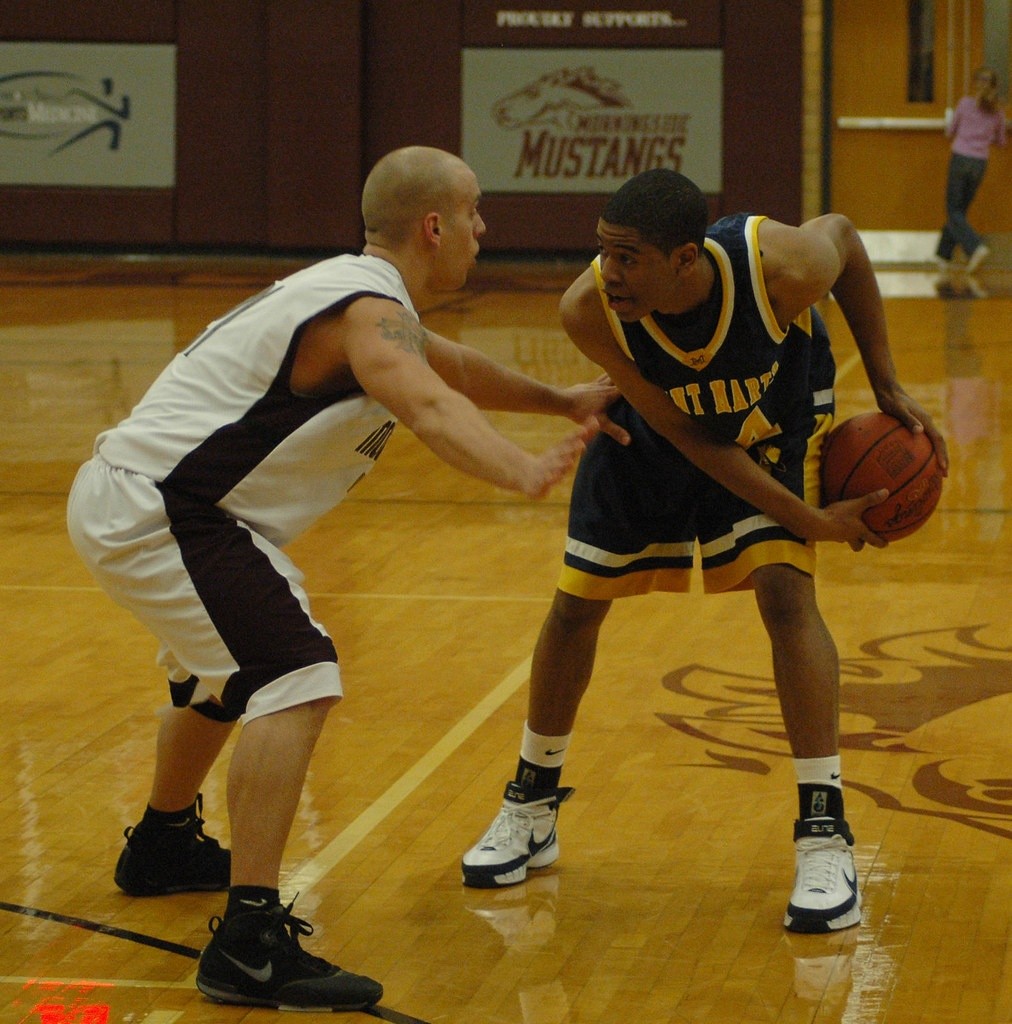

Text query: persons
(458, 168), (951, 933)
(922, 67), (1009, 274)
(65, 145), (630, 1013)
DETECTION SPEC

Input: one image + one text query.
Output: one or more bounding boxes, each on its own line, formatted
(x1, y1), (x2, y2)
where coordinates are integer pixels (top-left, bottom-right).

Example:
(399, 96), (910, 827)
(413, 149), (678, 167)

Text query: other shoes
(966, 247), (990, 273)
(931, 254), (957, 276)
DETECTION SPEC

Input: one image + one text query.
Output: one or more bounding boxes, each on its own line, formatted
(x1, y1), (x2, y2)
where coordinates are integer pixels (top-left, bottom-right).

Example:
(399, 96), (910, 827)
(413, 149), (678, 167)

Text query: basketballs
(818, 410), (944, 544)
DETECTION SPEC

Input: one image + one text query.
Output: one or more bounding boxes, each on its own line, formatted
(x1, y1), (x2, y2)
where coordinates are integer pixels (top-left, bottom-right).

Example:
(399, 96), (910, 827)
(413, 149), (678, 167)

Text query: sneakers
(783, 817), (862, 932)
(113, 816), (236, 896)
(196, 891), (385, 1010)
(459, 781), (576, 890)
